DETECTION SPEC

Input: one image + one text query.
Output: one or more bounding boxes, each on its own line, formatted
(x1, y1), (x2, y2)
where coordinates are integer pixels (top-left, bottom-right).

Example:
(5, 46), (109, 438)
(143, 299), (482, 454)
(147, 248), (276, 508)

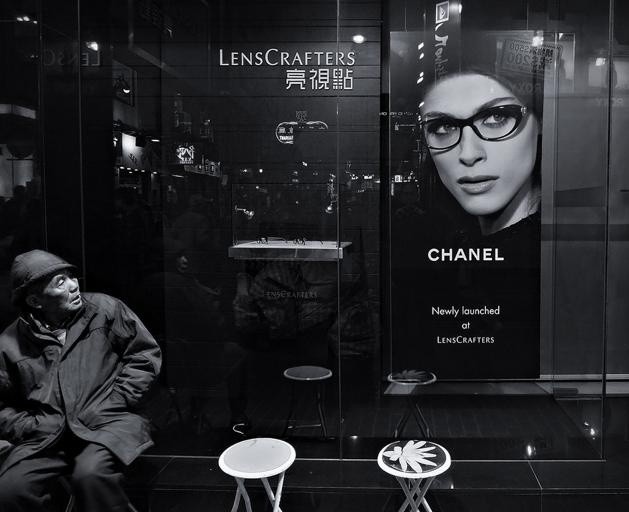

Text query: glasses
(416, 104), (531, 150)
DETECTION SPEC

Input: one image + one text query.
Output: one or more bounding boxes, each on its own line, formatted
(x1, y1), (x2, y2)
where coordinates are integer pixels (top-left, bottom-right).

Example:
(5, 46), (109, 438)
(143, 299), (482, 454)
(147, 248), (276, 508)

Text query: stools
(284, 366), (438, 440)
(377, 439), (453, 512)
(218, 436), (298, 512)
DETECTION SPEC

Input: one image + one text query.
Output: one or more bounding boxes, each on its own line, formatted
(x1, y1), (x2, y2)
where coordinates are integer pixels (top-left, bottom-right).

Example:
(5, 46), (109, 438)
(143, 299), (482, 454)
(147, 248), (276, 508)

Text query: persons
(0, 251), (162, 512)
(386, 67), (541, 381)
(116, 186), (251, 436)
(0, 181), (39, 263)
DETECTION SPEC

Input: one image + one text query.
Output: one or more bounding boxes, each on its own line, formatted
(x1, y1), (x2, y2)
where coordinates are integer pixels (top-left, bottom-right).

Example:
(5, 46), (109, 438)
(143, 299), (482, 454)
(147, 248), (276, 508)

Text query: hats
(417, 21), (544, 117)
